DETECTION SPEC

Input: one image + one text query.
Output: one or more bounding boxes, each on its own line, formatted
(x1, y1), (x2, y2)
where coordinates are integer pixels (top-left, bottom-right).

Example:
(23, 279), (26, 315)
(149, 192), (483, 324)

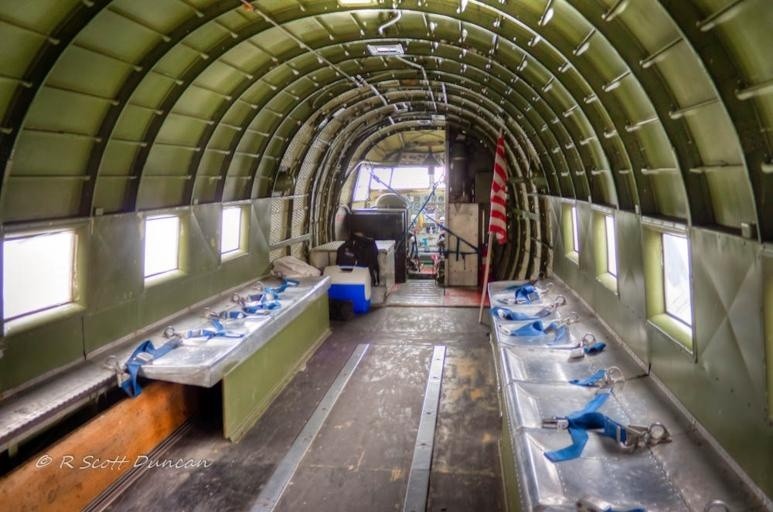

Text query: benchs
(95, 274), (332, 445)
(487, 275), (773, 512)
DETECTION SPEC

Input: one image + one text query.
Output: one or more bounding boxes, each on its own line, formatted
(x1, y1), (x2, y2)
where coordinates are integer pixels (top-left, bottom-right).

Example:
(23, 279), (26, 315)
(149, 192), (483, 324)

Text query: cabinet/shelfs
(311, 239), (396, 296)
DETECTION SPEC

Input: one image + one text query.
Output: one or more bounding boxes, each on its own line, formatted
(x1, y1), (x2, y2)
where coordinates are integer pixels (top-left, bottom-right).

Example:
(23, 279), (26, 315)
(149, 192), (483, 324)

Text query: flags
(488, 136), (509, 245)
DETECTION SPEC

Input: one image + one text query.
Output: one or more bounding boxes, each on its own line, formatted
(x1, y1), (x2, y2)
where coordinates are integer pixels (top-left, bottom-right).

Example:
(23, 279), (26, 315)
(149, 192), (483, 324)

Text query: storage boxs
(323, 265), (371, 313)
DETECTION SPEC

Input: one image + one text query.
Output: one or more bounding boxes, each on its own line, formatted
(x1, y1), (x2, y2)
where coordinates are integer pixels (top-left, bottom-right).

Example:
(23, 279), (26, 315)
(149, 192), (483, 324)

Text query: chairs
(370, 193), (408, 210)
